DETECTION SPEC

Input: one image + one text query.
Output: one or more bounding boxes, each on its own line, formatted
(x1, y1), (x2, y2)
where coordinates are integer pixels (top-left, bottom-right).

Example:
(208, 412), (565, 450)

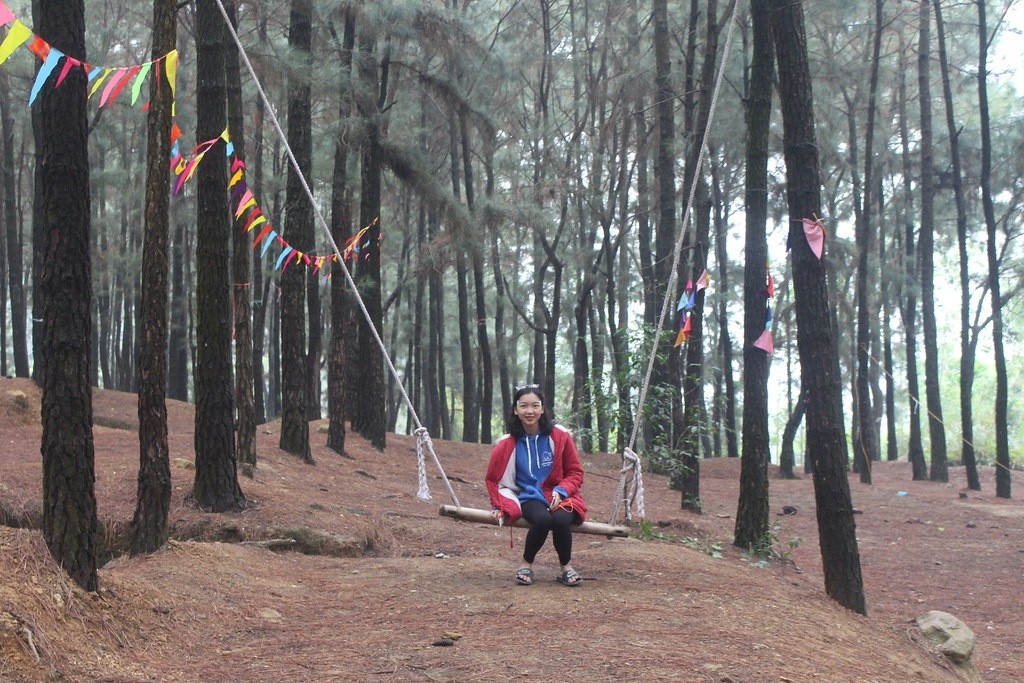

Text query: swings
(218, 2), (738, 537)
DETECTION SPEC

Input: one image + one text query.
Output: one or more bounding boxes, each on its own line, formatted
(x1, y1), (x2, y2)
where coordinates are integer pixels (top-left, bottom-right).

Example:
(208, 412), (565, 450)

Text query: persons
(486, 388), (587, 585)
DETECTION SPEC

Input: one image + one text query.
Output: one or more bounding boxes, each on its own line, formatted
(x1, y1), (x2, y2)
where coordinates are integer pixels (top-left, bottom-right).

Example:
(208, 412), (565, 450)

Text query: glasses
(515, 384), (541, 396)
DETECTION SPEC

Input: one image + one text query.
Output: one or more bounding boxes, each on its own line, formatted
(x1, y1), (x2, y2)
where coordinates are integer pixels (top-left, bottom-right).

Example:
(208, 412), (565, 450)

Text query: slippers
(556, 569), (583, 586)
(515, 567), (535, 584)
